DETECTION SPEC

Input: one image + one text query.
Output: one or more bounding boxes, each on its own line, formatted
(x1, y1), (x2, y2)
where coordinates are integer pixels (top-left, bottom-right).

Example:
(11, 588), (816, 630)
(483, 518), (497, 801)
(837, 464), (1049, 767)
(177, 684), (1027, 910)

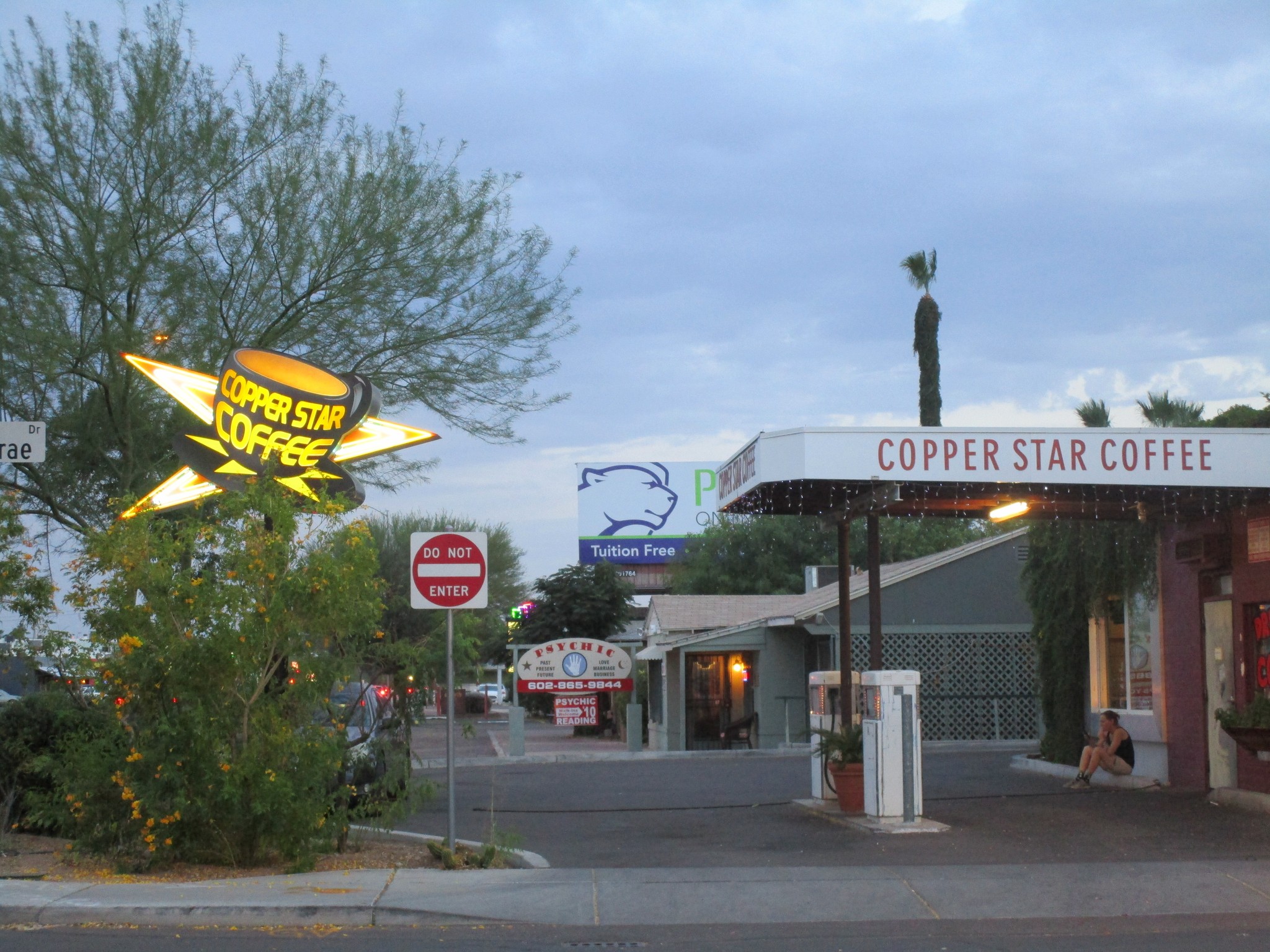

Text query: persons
(1061, 709), (1136, 789)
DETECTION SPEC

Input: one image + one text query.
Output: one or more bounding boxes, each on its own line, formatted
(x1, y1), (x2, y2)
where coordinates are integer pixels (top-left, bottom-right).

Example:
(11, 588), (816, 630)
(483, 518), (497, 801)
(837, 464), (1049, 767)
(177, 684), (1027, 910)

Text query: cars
(309, 675), (402, 820)
(471, 683), (507, 705)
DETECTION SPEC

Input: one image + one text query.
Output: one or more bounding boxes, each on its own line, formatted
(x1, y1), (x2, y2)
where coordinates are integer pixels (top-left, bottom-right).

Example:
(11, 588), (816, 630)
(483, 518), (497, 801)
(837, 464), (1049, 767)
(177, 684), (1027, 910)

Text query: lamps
(732, 655), (742, 671)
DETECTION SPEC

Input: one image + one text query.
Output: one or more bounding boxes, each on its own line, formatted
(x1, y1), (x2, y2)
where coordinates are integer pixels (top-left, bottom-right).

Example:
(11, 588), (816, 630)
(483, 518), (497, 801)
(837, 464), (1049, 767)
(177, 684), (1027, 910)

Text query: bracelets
(1099, 738), (1106, 743)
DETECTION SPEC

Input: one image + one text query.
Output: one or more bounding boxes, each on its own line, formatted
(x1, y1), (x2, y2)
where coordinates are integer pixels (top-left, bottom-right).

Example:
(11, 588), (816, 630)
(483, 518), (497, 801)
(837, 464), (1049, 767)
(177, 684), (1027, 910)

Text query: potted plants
(798, 720), (865, 812)
(1214, 688), (1270, 752)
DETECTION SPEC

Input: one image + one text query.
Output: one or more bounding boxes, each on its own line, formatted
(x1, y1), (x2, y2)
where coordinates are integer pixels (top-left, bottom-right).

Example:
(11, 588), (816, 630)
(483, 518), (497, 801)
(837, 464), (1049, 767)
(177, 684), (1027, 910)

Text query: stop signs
(409, 532), (489, 619)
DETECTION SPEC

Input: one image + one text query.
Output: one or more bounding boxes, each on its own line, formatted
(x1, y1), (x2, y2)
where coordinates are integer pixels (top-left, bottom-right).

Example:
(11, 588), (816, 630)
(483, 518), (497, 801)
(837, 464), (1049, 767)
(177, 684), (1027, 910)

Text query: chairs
(720, 713), (754, 750)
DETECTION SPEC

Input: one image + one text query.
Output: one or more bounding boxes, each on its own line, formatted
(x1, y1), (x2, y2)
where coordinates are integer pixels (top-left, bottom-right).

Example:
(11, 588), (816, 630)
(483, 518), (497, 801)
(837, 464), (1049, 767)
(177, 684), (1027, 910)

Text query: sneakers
(1071, 779), (1090, 790)
(1063, 778), (1082, 788)
